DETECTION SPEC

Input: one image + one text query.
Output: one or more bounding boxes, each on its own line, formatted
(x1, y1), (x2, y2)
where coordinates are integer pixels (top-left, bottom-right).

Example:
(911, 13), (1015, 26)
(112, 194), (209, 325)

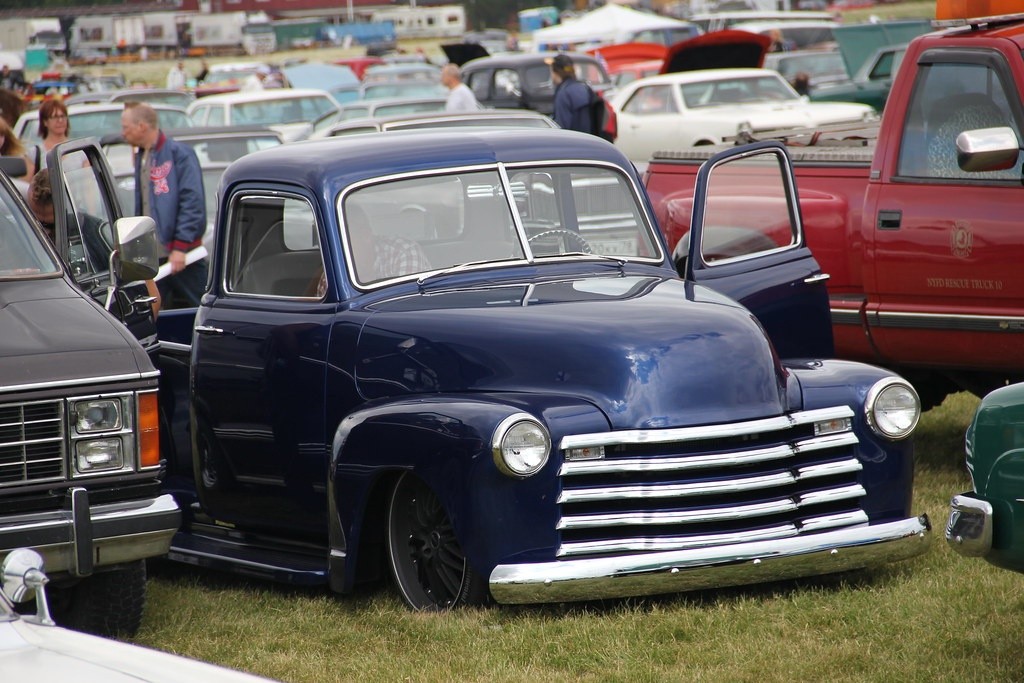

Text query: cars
(0, 13), (1024, 683)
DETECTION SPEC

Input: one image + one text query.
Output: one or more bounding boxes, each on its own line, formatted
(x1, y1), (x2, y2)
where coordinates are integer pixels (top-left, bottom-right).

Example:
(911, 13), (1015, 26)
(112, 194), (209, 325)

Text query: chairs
(242, 247), (328, 296)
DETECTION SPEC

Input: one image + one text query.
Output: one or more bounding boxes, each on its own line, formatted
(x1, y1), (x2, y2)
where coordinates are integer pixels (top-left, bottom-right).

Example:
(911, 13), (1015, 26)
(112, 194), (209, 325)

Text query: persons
(167, 57), (209, 91)
(551, 54), (591, 133)
(317, 202), (433, 298)
(121, 105), (207, 305)
(0, 99), (162, 322)
(442, 63), (477, 113)
(241, 65), (271, 89)
(505, 16), (576, 51)
(0, 65), (24, 93)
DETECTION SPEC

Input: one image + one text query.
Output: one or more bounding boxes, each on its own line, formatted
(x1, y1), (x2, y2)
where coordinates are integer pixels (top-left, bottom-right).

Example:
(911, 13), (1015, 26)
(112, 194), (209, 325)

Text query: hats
(552, 54), (572, 71)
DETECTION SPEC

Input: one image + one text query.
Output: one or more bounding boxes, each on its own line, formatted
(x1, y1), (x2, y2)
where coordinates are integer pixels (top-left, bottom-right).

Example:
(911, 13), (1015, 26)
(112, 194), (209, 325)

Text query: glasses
(48, 114), (67, 121)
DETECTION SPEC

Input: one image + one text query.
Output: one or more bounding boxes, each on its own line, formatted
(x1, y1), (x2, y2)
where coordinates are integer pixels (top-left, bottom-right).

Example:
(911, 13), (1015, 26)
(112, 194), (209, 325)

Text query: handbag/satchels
(587, 85), (617, 142)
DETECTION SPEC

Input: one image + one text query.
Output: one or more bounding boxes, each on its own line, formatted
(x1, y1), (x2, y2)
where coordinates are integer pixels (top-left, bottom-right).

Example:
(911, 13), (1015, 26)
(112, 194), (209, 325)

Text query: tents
(533, 3), (691, 58)
